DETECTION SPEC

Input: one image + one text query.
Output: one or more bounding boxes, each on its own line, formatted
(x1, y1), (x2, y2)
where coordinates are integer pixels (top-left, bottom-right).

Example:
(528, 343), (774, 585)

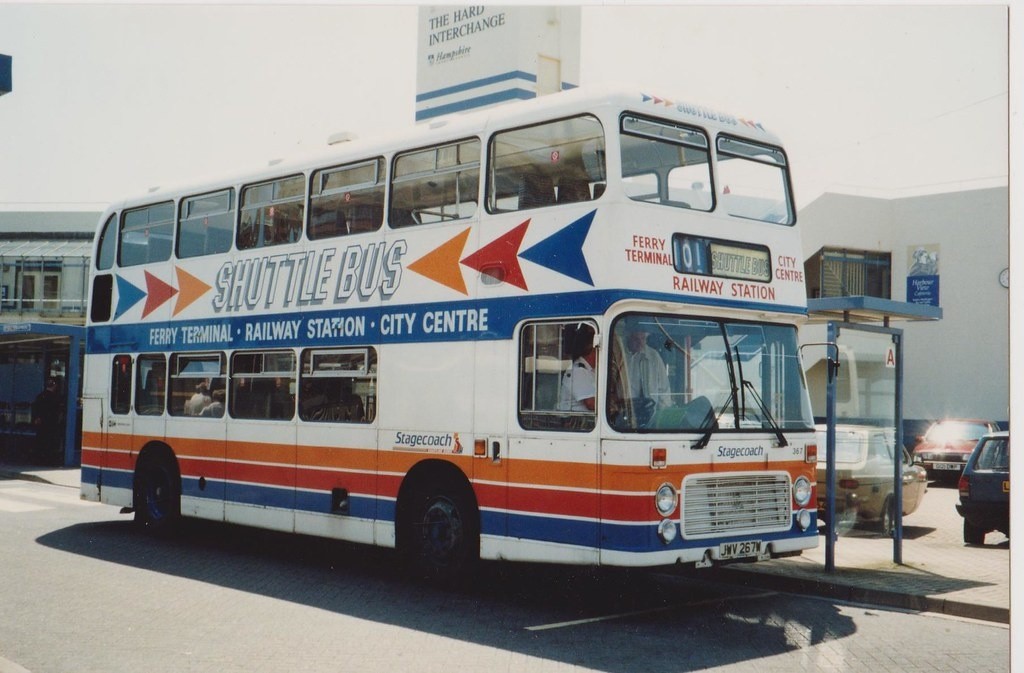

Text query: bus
(81, 81), (841, 597)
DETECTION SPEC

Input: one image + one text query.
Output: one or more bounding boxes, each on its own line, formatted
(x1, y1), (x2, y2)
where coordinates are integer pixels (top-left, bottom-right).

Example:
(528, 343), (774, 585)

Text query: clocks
(999, 268), (1008, 288)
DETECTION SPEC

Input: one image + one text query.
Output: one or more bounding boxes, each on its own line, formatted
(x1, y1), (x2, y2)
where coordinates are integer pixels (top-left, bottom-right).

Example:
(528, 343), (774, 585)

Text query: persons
(186, 382), (226, 419)
(552, 332), (596, 412)
(616, 331), (672, 407)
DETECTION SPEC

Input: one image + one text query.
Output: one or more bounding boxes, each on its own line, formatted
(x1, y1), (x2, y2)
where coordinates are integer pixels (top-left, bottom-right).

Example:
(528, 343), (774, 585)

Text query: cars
(955, 430), (1009, 544)
(813, 425), (928, 533)
(911, 419), (1001, 484)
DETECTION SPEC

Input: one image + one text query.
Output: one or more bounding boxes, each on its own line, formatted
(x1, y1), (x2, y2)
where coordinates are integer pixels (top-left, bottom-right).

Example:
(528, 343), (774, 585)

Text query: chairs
(515, 173), (694, 212)
(118, 204), (415, 269)
